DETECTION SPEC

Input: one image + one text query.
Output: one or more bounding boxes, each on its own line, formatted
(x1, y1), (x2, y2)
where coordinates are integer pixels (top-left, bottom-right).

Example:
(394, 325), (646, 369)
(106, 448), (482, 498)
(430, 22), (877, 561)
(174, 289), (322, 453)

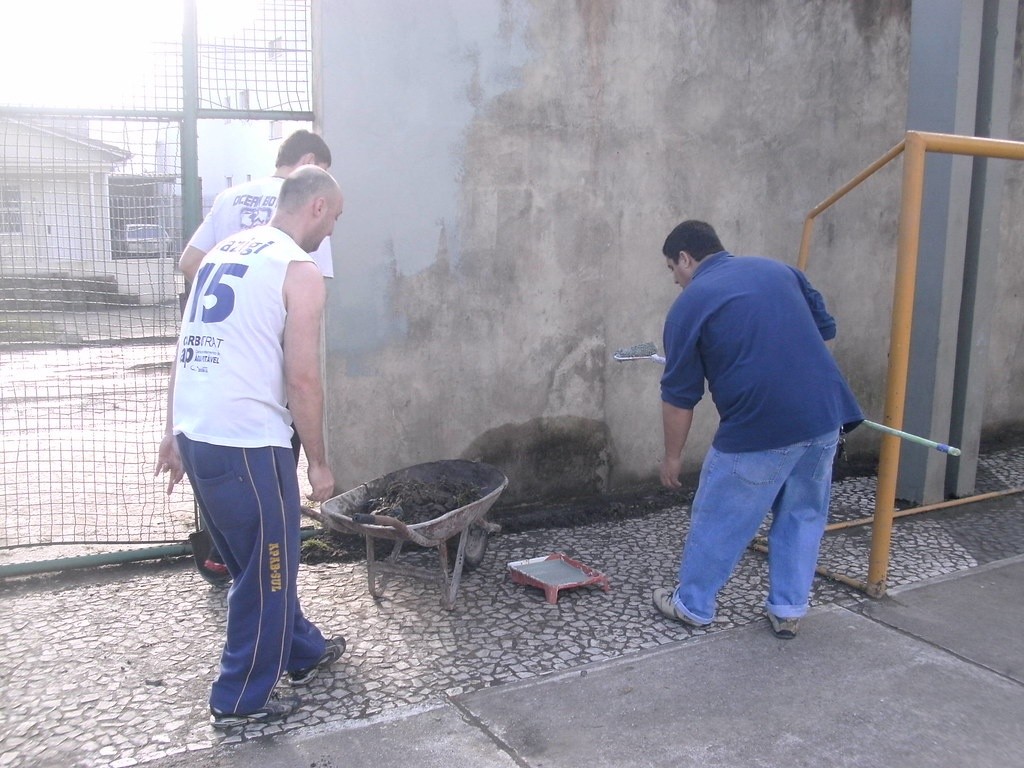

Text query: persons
(154, 164), (346, 728)
(653, 220), (864, 639)
(178, 126), (335, 582)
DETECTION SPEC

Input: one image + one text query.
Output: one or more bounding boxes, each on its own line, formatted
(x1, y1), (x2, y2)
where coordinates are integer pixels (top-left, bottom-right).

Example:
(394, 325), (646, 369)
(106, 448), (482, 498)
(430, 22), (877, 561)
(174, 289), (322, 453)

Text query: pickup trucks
(115, 223), (171, 257)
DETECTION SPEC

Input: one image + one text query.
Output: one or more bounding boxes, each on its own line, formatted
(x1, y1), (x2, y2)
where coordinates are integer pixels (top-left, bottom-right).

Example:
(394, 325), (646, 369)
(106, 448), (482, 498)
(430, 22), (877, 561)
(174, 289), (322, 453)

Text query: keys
(838, 424), (848, 461)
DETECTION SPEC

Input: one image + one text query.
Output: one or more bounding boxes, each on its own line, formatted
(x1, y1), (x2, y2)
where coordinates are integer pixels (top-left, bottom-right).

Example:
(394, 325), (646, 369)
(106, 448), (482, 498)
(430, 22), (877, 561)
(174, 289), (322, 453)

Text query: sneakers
(651, 584), (711, 628)
(209, 699), (293, 727)
(204, 546), (229, 576)
(287, 634), (345, 688)
(767, 610), (799, 640)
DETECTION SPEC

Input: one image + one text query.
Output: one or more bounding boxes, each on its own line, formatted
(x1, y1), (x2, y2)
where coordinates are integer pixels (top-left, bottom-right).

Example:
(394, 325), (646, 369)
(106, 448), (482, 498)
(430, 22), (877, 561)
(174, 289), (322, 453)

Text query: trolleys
(297, 457), (511, 609)
